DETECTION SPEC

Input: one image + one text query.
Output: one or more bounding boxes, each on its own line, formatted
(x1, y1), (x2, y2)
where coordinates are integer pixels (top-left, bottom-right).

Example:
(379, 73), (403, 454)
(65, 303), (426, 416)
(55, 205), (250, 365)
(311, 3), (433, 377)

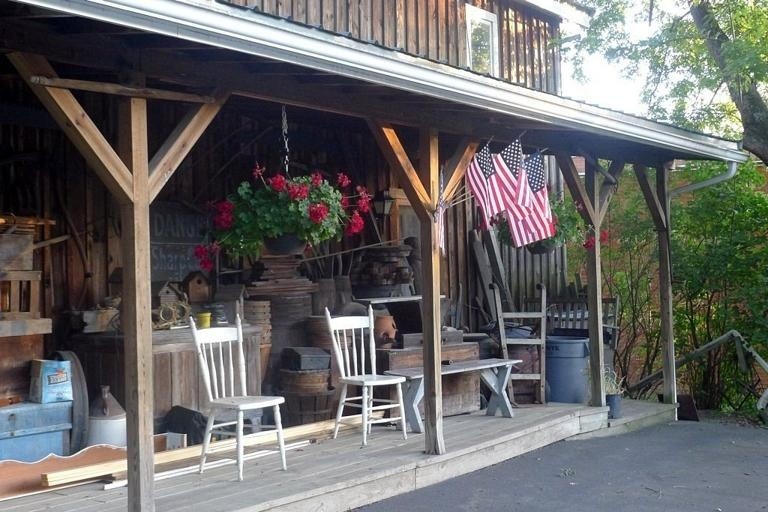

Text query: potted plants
(582, 363), (626, 420)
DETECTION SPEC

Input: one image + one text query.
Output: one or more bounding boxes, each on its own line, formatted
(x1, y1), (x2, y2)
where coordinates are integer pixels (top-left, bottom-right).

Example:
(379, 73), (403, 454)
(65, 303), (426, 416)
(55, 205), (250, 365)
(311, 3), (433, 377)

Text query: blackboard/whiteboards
(107, 198), (213, 284)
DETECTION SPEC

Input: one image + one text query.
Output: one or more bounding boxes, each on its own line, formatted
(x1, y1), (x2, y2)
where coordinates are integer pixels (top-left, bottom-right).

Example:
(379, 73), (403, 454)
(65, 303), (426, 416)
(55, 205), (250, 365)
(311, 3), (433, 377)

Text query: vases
(259, 227), (310, 259)
(526, 233), (559, 254)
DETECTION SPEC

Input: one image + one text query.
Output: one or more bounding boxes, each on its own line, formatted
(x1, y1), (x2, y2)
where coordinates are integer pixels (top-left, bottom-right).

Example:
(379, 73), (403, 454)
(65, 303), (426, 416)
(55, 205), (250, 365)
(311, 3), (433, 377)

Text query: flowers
(470, 191), (611, 249)
(196, 162), (372, 273)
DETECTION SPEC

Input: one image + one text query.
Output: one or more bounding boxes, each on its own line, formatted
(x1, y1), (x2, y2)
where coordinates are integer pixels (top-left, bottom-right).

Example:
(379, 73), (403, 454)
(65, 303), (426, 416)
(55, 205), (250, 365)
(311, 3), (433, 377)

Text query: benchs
(382, 357), (524, 434)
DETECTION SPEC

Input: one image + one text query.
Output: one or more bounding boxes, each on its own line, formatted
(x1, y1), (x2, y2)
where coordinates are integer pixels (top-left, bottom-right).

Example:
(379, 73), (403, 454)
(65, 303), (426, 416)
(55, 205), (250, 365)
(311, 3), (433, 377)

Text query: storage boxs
(29, 359), (73, 403)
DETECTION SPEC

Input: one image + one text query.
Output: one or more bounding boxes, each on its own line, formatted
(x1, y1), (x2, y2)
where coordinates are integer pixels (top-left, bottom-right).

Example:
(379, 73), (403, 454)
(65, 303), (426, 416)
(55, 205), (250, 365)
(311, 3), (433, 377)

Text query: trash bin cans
(545, 336), (591, 403)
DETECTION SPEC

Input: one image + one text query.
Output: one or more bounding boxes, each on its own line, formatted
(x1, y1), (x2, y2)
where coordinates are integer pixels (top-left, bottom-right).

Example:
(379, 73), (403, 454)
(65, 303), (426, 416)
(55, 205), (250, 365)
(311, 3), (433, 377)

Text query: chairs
(187, 317), (288, 478)
(326, 306), (407, 446)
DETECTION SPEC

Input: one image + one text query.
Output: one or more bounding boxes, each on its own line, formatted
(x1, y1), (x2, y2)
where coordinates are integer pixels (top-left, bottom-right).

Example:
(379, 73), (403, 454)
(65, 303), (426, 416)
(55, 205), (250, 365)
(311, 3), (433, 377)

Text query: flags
(464, 144), (509, 233)
(505, 150), (556, 248)
(493, 137), (534, 223)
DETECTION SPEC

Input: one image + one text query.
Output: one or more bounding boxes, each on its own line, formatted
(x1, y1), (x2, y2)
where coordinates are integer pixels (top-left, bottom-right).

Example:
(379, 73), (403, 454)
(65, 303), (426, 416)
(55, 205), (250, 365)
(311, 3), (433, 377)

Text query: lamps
(374, 190), (394, 218)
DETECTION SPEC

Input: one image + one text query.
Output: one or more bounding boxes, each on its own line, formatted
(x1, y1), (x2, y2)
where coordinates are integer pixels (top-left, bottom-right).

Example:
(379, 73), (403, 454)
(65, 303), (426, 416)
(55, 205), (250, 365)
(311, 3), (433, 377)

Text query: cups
(198, 313), (211, 328)
